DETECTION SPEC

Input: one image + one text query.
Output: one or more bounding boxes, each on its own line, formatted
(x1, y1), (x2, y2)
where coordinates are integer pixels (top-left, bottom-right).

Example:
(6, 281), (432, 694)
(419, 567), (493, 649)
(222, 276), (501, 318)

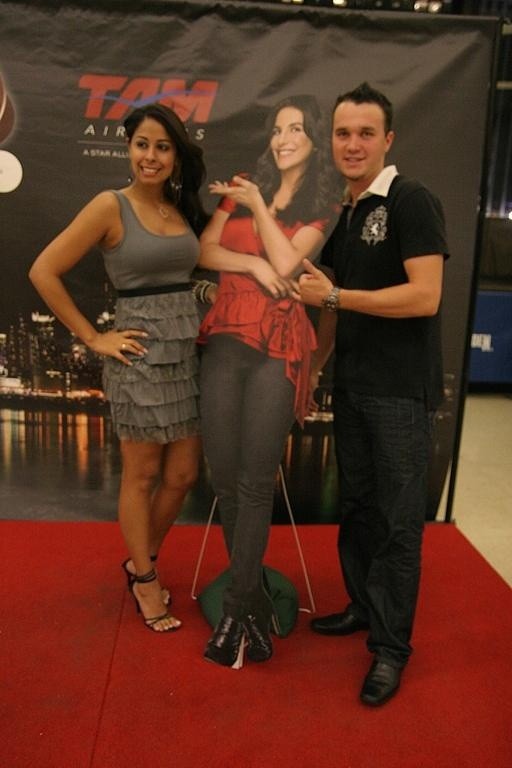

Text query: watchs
(322, 285), (340, 314)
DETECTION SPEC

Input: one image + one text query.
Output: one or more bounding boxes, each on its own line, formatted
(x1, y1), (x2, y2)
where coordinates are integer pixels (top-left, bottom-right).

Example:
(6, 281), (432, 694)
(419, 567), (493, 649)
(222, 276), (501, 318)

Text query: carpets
(0, 518), (512, 768)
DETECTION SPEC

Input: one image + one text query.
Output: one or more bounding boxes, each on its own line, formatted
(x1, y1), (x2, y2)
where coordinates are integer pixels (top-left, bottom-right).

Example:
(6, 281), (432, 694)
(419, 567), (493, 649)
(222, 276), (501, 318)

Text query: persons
(291, 82), (450, 705)
(28, 103), (217, 631)
(196, 91), (345, 669)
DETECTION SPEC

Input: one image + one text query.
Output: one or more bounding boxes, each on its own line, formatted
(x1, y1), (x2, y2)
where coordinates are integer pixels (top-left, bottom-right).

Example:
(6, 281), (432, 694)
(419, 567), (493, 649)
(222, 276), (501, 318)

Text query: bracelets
(191, 279), (213, 306)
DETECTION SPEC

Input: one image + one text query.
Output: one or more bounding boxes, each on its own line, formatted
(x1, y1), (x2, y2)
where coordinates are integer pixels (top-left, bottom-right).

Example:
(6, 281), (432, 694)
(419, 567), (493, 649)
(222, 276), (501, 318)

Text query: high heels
(245, 590), (283, 663)
(122, 553), (173, 608)
(128, 569), (177, 632)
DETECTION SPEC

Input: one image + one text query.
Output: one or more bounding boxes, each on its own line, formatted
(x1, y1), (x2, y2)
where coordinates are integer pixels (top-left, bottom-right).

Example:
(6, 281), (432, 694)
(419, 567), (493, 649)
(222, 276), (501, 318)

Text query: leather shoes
(311, 609), (369, 637)
(359, 659), (400, 707)
(203, 610), (244, 667)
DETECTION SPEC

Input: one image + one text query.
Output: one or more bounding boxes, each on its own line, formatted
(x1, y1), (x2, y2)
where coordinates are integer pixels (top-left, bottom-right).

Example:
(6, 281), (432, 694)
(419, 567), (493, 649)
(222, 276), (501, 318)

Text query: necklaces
(138, 192), (169, 220)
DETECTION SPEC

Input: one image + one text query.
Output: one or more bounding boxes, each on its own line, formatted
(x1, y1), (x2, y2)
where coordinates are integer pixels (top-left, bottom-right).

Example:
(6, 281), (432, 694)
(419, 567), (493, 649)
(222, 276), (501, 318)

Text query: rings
(122, 344), (126, 350)
